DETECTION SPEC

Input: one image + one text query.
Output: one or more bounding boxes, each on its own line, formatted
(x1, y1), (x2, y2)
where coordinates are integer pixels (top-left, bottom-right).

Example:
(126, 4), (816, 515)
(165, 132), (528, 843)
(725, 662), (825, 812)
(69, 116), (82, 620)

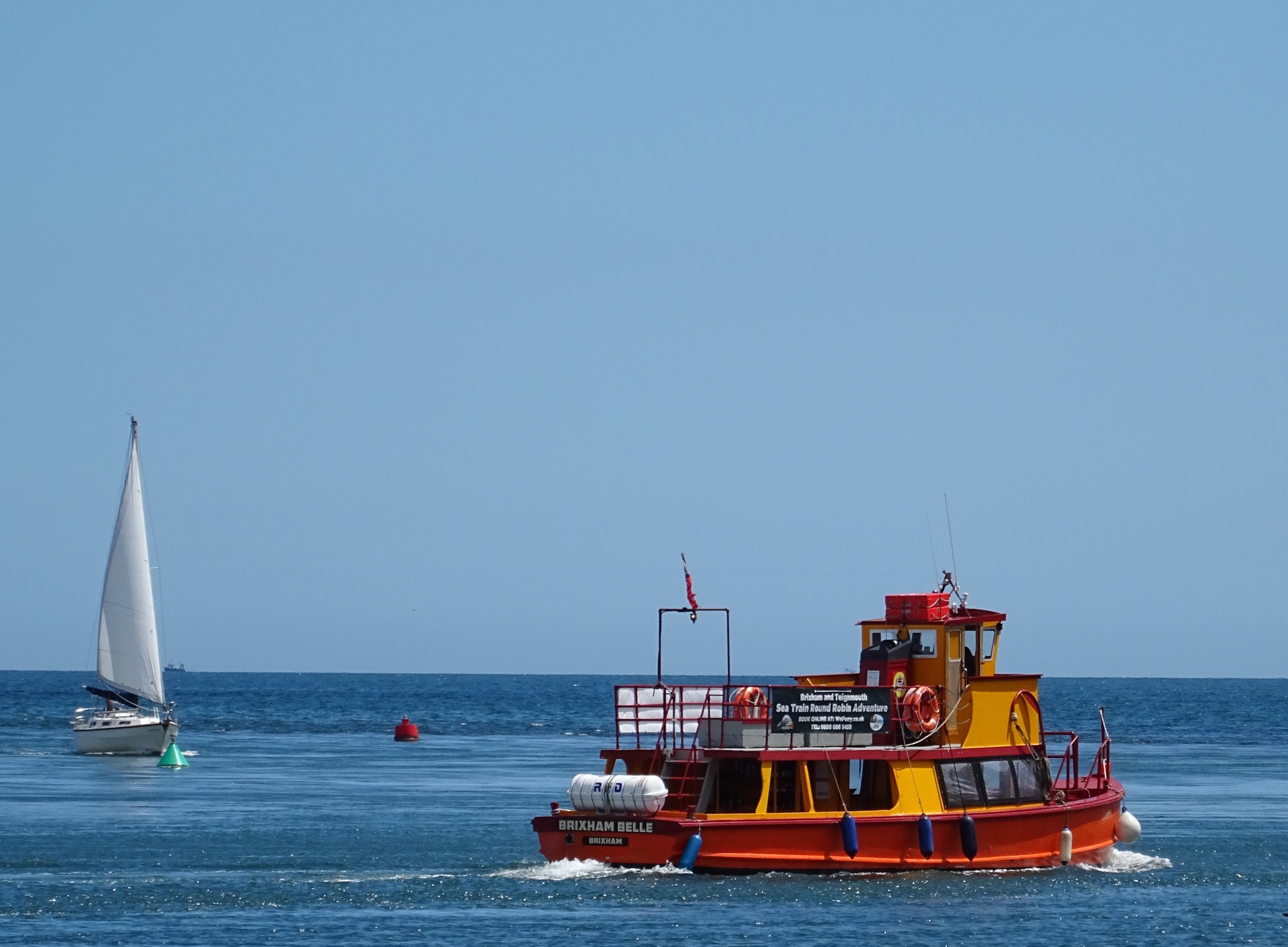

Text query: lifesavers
(740, 687), (768, 719)
(732, 687), (745, 720)
(903, 687), (925, 733)
(912, 684), (940, 733)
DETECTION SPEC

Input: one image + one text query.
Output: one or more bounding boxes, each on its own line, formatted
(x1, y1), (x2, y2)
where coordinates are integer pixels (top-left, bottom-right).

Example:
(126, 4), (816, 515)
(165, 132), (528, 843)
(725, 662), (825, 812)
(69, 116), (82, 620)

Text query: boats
(530, 553), (1143, 874)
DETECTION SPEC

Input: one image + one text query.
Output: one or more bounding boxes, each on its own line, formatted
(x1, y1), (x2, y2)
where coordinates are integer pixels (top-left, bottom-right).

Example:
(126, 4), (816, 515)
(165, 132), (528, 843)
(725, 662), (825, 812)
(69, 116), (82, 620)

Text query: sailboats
(68, 416), (189, 767)
